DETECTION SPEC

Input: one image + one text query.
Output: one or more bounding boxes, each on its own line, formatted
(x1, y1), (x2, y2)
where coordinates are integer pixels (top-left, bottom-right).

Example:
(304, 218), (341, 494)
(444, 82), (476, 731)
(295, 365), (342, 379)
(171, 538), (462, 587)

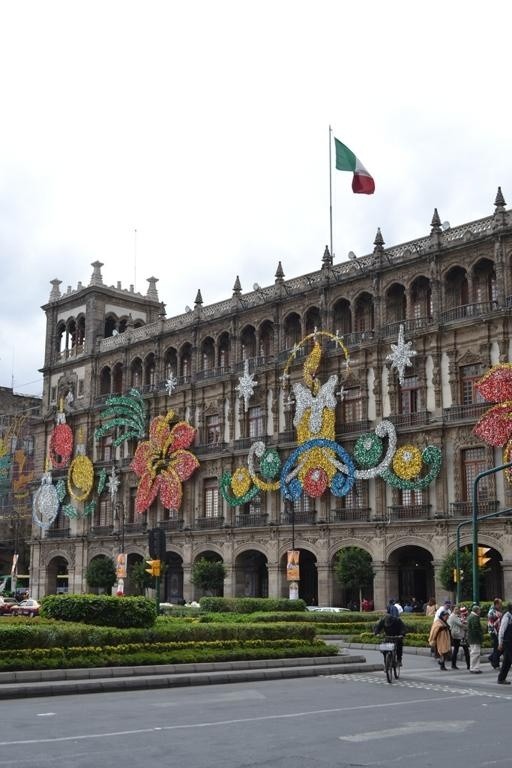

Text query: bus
(0, 575), (68, 595)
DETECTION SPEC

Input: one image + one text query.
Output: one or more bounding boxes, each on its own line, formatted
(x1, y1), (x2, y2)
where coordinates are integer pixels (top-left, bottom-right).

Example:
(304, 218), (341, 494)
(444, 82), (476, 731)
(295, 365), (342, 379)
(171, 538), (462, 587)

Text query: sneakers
(497, 679), (511, 684)
(488, 654), (501, 669)
(439, 662), (482, 674)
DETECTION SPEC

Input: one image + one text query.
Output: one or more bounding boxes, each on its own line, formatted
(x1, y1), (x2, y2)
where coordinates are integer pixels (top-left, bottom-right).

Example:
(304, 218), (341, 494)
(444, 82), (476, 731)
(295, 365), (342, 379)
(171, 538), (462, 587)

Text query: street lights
(284, 494), (295, 600)
(112, 501), (126, 597)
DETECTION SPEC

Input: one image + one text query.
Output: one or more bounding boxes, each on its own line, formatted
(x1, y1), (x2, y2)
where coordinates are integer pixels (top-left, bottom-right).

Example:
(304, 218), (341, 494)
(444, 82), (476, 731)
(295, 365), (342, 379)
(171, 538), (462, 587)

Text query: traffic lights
(478, 547), (491, 567)
(145, 560), (160, 576)
(460, 570), (464, 581)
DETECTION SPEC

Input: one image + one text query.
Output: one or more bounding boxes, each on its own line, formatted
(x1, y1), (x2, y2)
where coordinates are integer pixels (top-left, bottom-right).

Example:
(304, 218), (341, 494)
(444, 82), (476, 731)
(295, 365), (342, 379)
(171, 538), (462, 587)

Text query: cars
(309, 604), (350, 614)
(0, 595), (42, 617)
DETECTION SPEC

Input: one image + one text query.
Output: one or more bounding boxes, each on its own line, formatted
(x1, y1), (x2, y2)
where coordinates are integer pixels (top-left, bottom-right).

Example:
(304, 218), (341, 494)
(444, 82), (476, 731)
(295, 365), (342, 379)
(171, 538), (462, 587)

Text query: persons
(361, 598), (372, 612)
(374, 597), (512, 685)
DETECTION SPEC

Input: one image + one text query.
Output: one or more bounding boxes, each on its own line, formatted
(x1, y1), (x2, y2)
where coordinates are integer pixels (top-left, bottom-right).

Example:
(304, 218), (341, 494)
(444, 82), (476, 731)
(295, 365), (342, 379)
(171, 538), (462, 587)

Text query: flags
(334, 137), (375, 195)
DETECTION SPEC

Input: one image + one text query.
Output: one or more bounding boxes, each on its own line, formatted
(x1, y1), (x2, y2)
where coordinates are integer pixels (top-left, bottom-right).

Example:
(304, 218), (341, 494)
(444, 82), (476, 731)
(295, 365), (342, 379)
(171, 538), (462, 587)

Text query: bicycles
(377, 631), (405, 684)
